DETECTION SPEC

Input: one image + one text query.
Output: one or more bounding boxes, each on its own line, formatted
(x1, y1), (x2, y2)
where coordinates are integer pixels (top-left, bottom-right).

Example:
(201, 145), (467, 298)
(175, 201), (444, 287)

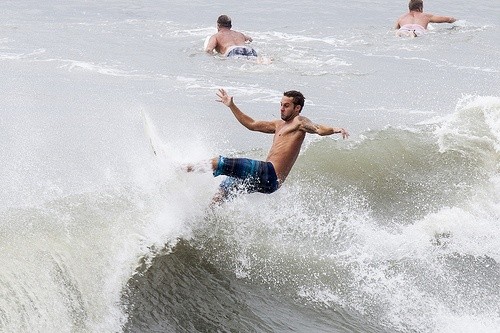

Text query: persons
(172, 86), (349, 209)
(394, 0), (459, 39)
(205, 13), (258, 59)
